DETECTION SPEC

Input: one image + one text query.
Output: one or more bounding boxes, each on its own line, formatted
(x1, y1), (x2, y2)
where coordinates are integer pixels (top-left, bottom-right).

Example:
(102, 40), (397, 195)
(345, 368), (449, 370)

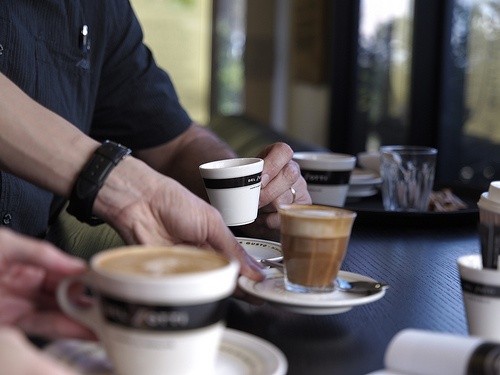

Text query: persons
(0, 0), (314, 263)
(0, 75), (270, 375)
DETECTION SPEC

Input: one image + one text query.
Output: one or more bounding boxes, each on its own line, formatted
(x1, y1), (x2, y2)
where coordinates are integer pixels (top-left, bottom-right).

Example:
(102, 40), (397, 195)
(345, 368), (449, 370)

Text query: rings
(289, 187), (296, 203)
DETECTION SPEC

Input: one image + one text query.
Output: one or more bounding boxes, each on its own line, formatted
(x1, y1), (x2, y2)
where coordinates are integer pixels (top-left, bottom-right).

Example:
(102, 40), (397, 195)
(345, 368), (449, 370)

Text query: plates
(42, 328), (287, 375)
(234, 237), (282, 262)
(238, 266), (385, 314)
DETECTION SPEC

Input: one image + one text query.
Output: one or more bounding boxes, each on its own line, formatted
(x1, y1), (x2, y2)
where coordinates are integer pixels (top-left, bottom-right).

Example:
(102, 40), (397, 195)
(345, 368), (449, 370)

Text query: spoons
(262, 258), (382, 293)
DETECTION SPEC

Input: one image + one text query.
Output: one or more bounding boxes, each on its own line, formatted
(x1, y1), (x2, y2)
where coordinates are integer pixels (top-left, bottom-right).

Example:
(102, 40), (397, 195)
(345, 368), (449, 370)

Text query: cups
(384, 328), (500, 375)
(380, 146), (437, 211)
(292, 152), (356, 206)
(57, 245), (240, 375)
(199, 158), (264, 226)
(458, 255), (500, 334)
(477, 181), (500, 269)
(279, 205), (355, 286)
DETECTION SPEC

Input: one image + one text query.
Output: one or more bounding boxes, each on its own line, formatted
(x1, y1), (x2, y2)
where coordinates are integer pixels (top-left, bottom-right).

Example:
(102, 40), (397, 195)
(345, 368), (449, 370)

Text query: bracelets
(66, 140), (133, 228)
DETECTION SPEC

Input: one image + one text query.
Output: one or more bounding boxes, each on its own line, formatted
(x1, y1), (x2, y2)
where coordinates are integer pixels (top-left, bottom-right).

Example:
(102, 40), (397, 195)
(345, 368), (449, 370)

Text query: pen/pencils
(78, 22), (93, 59)
